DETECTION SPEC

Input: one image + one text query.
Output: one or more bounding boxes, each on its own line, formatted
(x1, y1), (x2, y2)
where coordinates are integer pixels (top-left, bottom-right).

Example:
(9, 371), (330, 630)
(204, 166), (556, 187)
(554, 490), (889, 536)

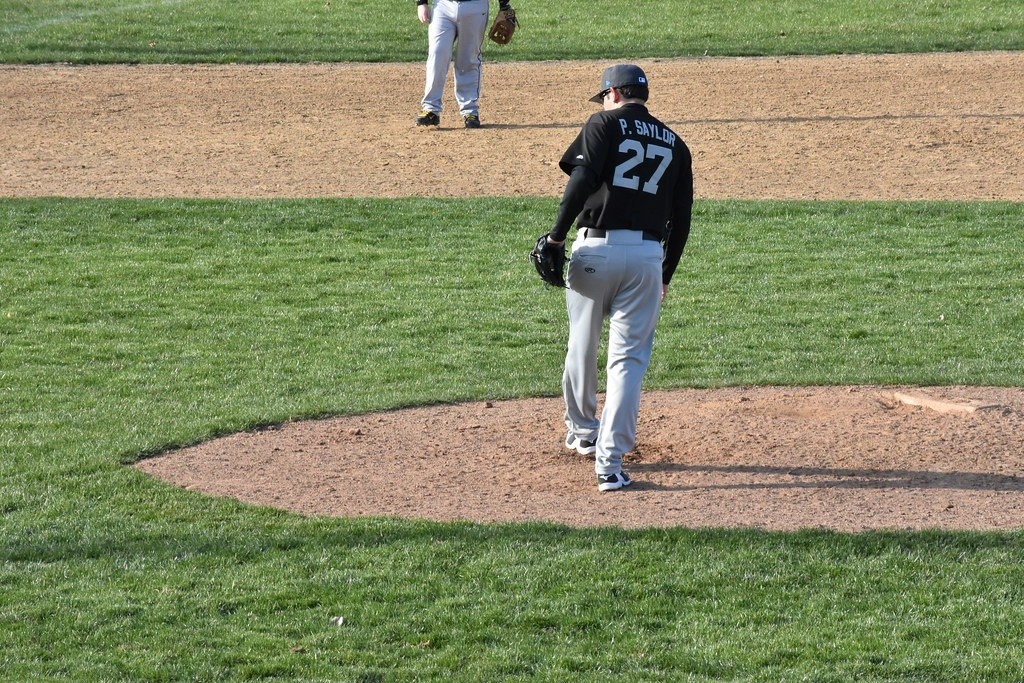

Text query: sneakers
(415, 110), (439, 127)
(597, 470), (631, 492)
(565, 431), (597, 455)
(464, 114), (480, 127)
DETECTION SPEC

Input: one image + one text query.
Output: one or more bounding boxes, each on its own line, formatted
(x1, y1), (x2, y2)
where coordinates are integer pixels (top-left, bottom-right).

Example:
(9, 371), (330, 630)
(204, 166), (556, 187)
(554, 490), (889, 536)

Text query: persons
(415, 0), (516, 129)
(529, 65), (693, 492)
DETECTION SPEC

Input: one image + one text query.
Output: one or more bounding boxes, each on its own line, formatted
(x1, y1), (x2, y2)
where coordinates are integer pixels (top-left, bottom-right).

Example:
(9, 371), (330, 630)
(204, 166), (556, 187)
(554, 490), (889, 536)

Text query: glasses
(600, 87), (621, 100)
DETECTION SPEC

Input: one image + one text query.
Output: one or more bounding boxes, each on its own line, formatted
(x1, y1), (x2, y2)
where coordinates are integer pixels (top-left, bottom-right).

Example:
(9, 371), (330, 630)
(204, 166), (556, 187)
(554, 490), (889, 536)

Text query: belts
(585, 228), (662, 243)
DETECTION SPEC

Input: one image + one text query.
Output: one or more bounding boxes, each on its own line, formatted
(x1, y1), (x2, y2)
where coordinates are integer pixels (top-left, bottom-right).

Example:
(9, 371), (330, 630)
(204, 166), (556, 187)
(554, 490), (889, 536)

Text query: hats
(589, 64), (648, 104)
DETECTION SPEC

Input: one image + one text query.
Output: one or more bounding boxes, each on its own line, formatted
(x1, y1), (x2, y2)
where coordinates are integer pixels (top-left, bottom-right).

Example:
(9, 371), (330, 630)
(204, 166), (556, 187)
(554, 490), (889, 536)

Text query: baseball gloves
(489, 9), (518, 45)
(530, 234), (568, 288)
